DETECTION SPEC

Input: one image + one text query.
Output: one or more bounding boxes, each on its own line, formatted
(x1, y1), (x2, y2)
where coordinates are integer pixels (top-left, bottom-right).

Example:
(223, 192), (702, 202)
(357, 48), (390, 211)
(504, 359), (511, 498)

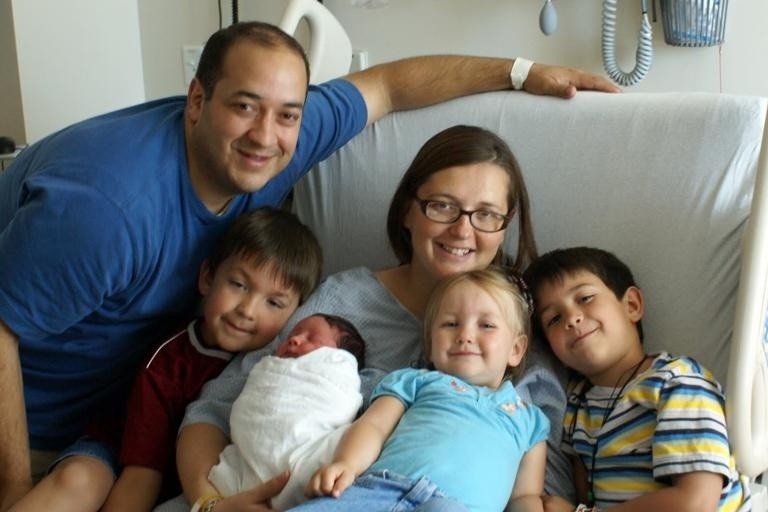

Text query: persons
(287, 267), (552, 512)
(523, 245), (752, 512)
(206, 312), (367, 509)
(153, 125), (576, 512)
(0, 20), (625, 512)
(1, 203), (322, 512)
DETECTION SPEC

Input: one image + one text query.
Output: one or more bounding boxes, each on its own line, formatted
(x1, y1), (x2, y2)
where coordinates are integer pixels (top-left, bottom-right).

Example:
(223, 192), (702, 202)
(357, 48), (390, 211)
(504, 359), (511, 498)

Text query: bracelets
(188, 490), (222, 512)
(507, 54), (536, 91)
(572, 503), (600, 512)
(202, 495), (221, 512)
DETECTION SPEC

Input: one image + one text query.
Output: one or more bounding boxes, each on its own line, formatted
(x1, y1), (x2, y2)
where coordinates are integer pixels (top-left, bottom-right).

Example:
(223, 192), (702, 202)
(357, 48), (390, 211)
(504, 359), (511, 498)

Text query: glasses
(415, 196), (510, 232)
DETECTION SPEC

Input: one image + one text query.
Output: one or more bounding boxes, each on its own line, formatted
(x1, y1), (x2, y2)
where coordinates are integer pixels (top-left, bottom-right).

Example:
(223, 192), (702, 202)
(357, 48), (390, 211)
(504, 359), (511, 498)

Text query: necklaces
(569, 353), (649, 507)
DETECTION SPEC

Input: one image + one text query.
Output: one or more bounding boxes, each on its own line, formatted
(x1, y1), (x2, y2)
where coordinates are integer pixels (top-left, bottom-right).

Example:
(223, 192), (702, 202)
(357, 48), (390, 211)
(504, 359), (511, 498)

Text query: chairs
(294, 88), (768, 512)
